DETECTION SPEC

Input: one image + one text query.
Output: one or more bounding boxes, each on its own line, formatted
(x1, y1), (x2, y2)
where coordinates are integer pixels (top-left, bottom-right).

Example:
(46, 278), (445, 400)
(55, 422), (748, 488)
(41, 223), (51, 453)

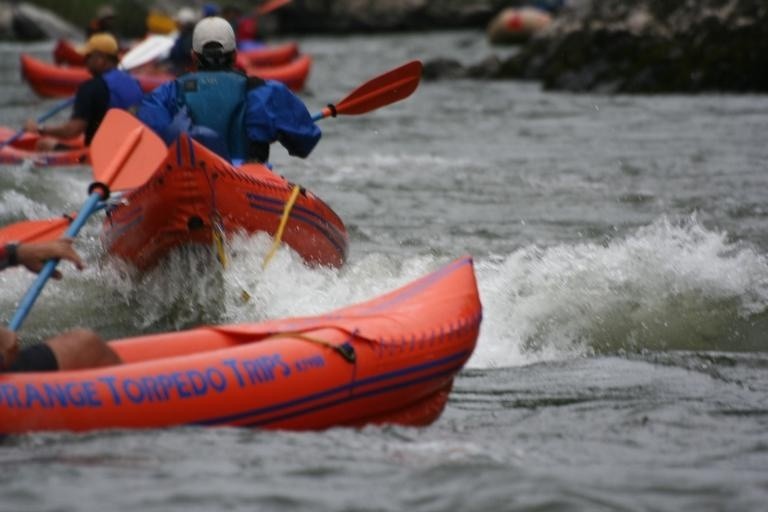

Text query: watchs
(5, 240), (20, 265)
(37, 124), (44, 136)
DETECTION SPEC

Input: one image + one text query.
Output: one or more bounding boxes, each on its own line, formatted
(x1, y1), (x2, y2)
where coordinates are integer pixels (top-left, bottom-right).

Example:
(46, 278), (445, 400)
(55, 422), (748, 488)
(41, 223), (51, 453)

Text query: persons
(0, 237), (128, 374)
(25, 32), (146, 150)
(138, 15), (322, 170)
(86, 6), (264, 77)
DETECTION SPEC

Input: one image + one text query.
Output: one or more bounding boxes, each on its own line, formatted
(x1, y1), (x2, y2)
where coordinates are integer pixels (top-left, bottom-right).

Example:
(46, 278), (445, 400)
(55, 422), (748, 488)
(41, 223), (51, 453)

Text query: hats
(192, 16), (235, 54)
(74, 34), (118, 56)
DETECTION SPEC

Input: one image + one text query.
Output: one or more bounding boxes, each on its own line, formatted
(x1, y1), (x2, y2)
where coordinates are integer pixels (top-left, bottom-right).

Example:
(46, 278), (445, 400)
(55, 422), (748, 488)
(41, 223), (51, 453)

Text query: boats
(102, 131), (348, 275)
(1, 255), (485, 435)
(489, 6), (550, 47)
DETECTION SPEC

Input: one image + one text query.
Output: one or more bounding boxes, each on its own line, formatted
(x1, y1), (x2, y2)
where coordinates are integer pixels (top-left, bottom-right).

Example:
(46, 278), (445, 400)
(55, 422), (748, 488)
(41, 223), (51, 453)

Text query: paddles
(5, 35), (175, 144)
(312, 61), (422, 121)
(4, 107), (169, 327)
